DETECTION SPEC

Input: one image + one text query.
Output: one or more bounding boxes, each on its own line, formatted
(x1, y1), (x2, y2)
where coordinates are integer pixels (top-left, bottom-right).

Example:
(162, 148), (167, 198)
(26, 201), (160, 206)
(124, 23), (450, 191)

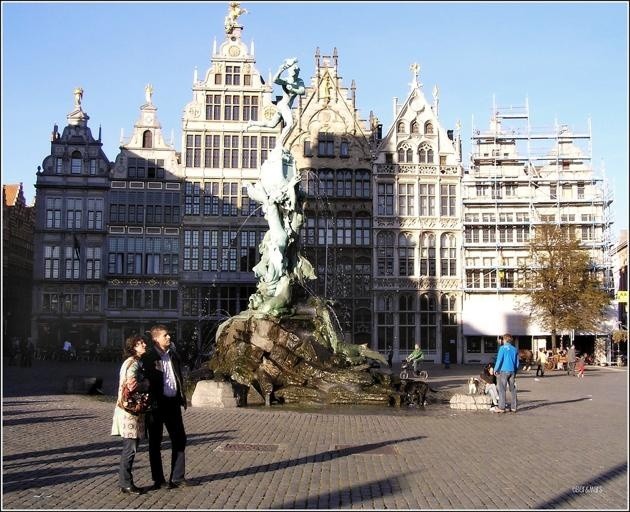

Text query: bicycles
(399, 356), (429, 380)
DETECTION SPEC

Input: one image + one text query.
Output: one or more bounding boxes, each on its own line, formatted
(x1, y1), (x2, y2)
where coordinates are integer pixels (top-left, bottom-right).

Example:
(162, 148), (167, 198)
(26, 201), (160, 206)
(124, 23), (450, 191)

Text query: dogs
(468, 376), (478, 395)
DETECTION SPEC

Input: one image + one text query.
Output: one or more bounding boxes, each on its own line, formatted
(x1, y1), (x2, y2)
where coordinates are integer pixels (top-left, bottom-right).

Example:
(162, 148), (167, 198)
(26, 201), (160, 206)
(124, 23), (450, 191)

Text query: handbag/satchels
(117, 383), (151, 415)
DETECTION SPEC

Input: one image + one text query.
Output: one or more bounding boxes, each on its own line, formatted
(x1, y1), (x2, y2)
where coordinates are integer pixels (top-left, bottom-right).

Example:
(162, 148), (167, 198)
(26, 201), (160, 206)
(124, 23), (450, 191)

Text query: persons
(9, 335), (93, 368)
(493, 333), (520, 413)
(386, 344), (393, 368)
(142, 324), (200, 488)
(406, 343), (424, 375)
(480, 363), (508, 410)
(243, 55), (306, 154)
(522, 344), (588, 378)
(109, 331), (149, 496)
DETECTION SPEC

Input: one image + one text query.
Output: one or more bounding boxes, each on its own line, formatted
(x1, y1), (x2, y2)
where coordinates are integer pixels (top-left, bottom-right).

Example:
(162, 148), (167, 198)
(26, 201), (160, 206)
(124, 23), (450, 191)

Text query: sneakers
(494, 406), (505, 413)
(511, 409), (516, 412)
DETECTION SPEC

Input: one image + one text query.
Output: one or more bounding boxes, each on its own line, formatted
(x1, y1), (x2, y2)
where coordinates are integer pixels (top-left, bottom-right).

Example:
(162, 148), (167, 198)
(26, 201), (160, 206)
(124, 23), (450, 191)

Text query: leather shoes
(169, 479), (199, 486)
(154, 482), (173, 489)
(119, 486), (142, 495)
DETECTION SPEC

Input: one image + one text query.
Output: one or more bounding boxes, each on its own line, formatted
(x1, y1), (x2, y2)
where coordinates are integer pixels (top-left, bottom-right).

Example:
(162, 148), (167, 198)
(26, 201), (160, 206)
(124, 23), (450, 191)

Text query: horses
(518, 349), (534, 371)
